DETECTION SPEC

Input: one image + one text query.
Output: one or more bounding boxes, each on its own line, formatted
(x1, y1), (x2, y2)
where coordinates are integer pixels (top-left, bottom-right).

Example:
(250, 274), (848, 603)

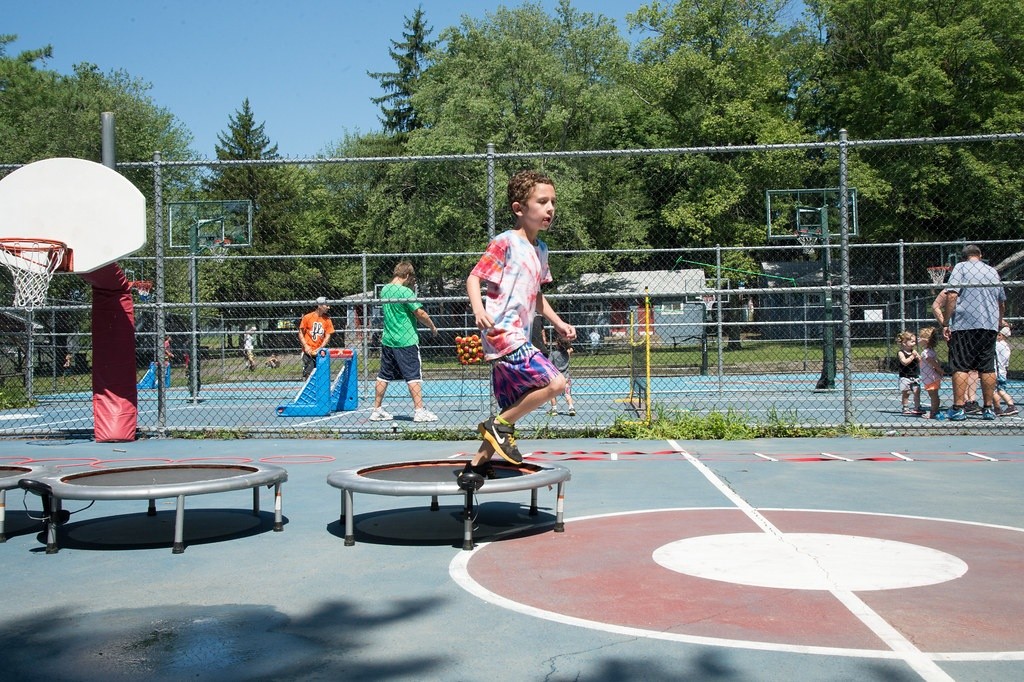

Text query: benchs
(670, 333), (717, 348)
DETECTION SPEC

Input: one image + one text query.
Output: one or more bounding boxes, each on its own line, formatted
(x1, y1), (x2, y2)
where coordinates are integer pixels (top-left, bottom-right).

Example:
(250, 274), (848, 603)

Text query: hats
(316, 296), (328, 301)
(999, 326), (1011, 338)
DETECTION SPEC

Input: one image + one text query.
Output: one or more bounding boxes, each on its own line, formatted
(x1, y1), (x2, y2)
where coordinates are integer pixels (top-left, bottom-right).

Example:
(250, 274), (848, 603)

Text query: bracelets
(941, 324), (949, 327)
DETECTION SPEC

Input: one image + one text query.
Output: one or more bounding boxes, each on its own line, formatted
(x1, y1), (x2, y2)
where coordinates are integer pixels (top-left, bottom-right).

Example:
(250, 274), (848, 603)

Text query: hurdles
(136, 361), (171, 389)
(614, 286), (652, 425)
(276, 347), (358, 417)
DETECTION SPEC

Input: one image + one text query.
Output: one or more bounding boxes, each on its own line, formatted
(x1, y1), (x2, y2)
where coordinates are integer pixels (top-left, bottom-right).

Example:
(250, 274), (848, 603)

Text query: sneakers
(547, 409), (558, 416)
(982, 407), (996, 420)
(963, 401), (983, 414)
(369, 407), (393, 421)
(946, 405), (967, 421)
(478, 415), (523, 462)
(413, 409), (440, 422)
(569, 408), (575, 416)
(458, 462), (500, 481)
(994, 406), (1019, 416)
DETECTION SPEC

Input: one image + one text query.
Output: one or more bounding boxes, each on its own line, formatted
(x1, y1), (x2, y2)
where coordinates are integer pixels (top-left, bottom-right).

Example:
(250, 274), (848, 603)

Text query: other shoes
(902, 408), (926, 414)
(921, 411), (940, 419)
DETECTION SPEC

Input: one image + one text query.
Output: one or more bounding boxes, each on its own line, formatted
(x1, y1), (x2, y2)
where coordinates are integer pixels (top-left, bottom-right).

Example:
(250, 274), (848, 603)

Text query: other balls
(312, 351), (316, 356)
(455, 334), (484, 365)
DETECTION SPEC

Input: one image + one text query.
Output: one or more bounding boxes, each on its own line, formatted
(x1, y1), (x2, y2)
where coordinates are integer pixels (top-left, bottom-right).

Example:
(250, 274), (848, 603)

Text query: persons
(896, 244), (1018, 420)
(299, 296), (335, 381)
(164, 326), (280, 374)
(369, 261), (441, 421)
(465, 167), (599, 479)
